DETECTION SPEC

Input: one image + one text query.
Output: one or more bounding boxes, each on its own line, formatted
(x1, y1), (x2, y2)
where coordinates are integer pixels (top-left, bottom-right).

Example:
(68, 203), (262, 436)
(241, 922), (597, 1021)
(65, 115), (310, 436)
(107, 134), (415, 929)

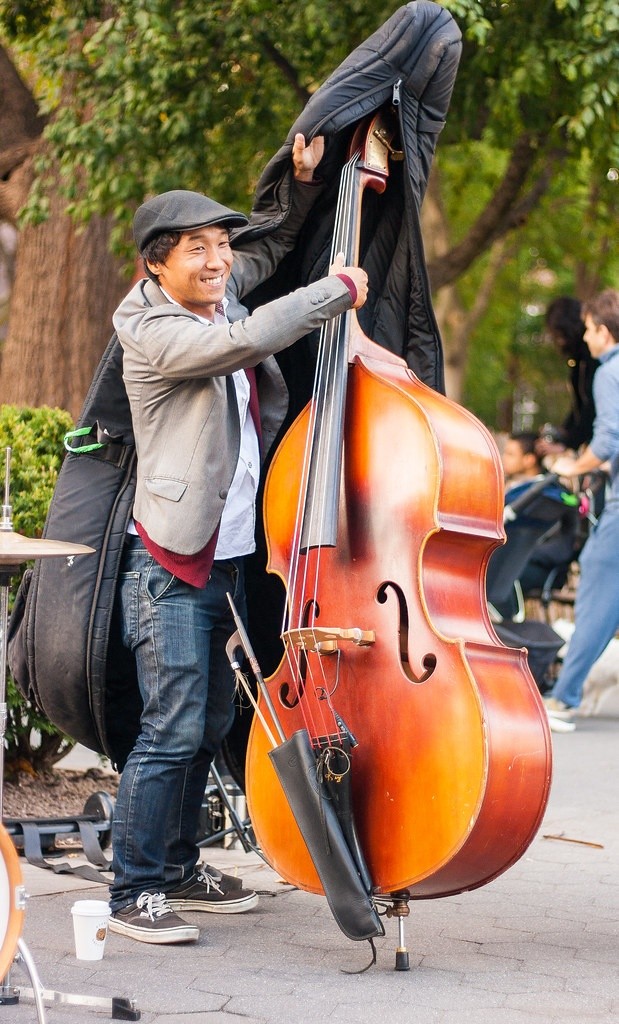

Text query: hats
(133, 190), (248, 254)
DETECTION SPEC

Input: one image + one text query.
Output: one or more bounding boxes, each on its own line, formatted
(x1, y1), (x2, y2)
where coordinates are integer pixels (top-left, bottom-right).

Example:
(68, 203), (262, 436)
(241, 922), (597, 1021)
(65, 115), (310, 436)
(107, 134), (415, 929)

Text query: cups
(71, 900), (111, 960)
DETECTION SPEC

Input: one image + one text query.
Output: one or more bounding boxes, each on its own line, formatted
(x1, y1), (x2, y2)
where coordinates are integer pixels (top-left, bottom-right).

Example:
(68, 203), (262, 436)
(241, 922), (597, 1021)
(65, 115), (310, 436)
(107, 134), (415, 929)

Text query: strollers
(483, 462), (593, 687)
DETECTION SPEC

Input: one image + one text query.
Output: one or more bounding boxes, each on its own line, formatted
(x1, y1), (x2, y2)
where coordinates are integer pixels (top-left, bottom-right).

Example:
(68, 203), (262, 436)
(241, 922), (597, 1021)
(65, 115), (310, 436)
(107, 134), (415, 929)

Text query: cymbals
(0, 525), (102, 569)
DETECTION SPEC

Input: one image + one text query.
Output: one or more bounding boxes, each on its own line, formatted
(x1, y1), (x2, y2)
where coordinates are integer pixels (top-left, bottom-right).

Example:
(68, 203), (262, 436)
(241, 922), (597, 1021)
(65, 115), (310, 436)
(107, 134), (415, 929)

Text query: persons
(500, 283), (619, 735)
(108, 130), (367, 947)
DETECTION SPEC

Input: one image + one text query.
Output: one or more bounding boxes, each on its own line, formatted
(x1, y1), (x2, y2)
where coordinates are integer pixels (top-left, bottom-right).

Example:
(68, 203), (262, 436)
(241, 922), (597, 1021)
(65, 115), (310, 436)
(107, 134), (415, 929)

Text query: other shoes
(542, 698), (576, 732)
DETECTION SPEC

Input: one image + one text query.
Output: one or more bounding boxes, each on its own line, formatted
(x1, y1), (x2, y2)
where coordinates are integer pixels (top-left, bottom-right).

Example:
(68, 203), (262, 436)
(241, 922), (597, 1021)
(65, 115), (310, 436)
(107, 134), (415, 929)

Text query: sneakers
(162, 862), (258, 914)
(105, 892), (197, 944)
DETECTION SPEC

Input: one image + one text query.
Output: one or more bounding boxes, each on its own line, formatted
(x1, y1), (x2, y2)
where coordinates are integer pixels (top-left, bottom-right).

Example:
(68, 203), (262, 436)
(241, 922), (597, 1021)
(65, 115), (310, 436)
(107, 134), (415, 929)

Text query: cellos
(243, 107), (552, 972)
